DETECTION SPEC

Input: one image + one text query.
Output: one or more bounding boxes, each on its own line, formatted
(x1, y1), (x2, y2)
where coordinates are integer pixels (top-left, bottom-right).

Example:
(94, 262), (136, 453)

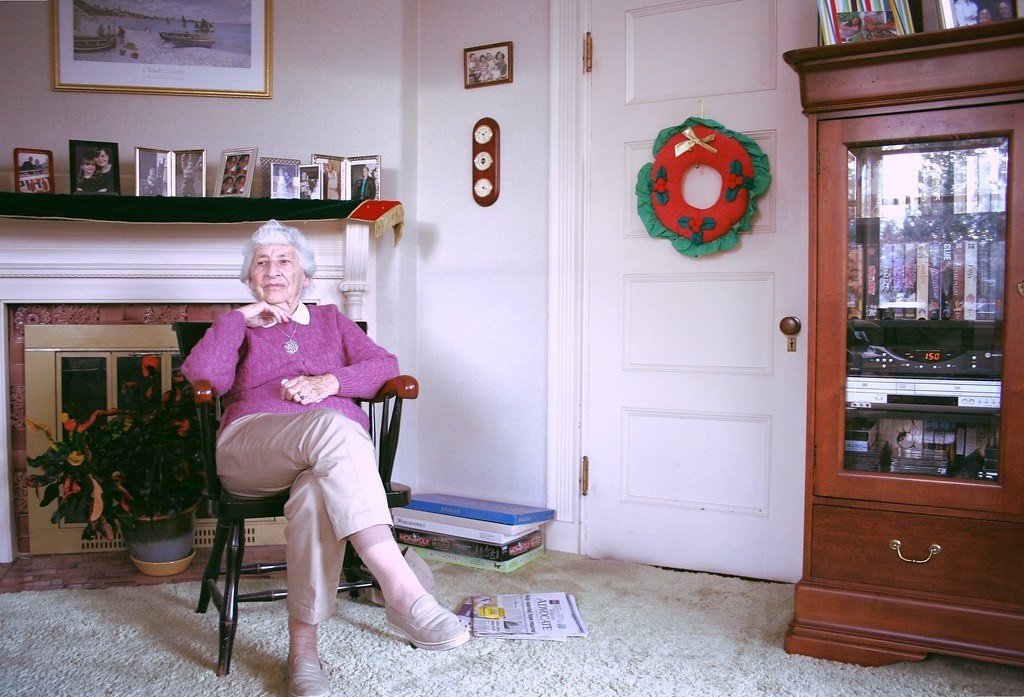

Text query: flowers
(20, 356), (220, 542)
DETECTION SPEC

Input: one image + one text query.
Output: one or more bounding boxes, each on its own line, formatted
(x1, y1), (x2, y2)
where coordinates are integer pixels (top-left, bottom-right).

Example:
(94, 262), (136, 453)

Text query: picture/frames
(134, 147), (207, 198)
(14, 148), (55, 194)
(463, 41), (513, 89)
(69, 139), (121, 196)
(50, 0), (274, 99)
(936, 0), (1019, 30)
(214, 147), (259, 198)
(260, 152), (381, 201)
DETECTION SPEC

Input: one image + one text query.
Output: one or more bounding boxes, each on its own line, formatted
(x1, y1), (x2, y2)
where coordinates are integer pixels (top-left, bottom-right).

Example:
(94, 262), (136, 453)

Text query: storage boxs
(391, 493), (556, 573)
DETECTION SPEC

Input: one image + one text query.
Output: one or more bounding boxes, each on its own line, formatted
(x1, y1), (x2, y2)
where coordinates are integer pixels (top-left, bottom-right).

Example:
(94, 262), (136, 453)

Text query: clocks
(472, 117), (499, 207)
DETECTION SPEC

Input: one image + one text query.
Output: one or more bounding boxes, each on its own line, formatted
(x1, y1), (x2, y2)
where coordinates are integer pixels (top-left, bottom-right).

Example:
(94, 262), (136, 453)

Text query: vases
(117, 496), (202, 564)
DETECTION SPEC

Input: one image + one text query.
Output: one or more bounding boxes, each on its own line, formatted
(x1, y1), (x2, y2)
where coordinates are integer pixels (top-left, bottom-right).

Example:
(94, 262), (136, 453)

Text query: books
(847, 240), (1005, 321)
(861, 145), (1007, 216)
(843, 418), (999, 481)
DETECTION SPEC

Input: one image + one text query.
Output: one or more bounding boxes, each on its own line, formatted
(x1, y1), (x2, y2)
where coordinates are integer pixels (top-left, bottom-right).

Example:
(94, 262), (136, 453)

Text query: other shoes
(288, 652), (330, 697)
(385, 592), (469, 652)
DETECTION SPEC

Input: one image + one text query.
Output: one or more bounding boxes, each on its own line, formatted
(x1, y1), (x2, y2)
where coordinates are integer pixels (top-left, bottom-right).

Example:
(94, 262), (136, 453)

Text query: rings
(297, 392), (305, 400)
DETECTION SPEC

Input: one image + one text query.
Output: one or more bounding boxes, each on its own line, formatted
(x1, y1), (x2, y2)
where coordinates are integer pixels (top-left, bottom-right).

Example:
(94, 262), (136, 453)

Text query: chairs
(171, 320), (419, 676)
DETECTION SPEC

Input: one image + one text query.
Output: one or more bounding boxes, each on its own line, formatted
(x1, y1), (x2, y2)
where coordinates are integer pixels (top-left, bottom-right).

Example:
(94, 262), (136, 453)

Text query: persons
(97, 23), (126, 44)
(181, 221), (471, 697)
(21, 157), (43, 175)
(30, 178), (48, 192)
(76, 146), (116, 194)
(180, 154), (202, 197)
(469, 51), (508, 83)
(839, 11), (897, 41)
(323, 165), (339, 200)
(358, 168), (375, 200)
(284, 171), (294, 193)
(146, 168), (162, 195)
(221, 156), (249, 194)
(955, 0), (1014, 27)
(300, 171), (314, 199)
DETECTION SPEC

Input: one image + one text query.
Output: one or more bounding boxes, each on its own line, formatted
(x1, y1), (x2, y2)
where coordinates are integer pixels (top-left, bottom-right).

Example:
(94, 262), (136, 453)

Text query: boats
(74, 32), (117, 54)
(158, 30), (201, 43)
(170, 34), (217, 49)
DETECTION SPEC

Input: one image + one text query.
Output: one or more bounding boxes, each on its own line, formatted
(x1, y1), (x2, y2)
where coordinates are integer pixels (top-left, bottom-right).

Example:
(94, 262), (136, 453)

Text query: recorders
(847, 318), (1003, 380)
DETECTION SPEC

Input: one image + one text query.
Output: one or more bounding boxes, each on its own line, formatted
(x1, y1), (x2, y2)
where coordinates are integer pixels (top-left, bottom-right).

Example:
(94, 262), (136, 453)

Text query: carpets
(1, 548), (1024, 696)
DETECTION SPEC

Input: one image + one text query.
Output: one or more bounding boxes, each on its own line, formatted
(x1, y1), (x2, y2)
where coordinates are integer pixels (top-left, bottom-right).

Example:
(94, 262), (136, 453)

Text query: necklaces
(274, 321), (300, 354)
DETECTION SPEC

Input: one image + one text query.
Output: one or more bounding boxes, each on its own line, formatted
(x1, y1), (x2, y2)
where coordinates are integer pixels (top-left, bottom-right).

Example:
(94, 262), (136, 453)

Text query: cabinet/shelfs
(780, 16), (1024, 668)
